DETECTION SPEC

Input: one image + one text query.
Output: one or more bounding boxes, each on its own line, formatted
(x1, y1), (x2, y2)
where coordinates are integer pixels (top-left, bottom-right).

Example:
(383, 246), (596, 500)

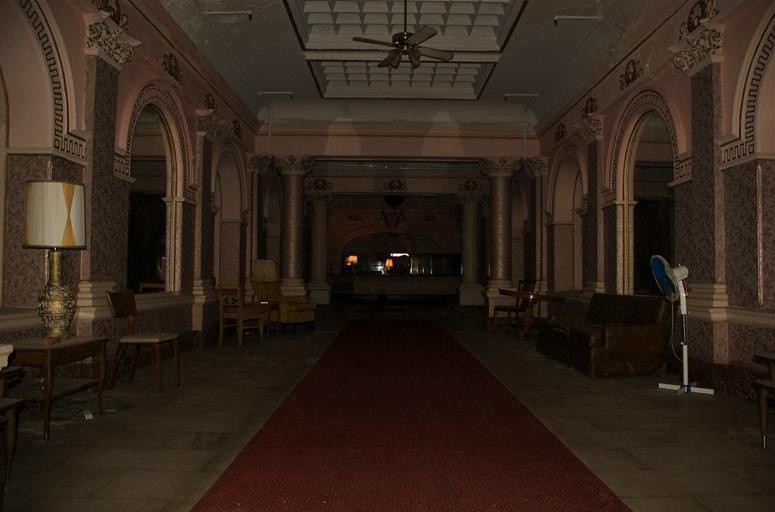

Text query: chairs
(214, 282), (317, 351)
(101, 286), (180, 395)
(491, 277), (537, 328)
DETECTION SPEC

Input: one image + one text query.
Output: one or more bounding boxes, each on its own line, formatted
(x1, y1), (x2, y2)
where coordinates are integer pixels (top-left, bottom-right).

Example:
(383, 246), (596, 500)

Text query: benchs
(538, 290), (678, 381)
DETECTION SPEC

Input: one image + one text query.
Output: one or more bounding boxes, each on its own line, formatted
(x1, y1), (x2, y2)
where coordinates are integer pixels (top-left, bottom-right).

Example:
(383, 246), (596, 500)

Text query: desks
(499, 287), (565, 342)
(6, 334), (109, 441)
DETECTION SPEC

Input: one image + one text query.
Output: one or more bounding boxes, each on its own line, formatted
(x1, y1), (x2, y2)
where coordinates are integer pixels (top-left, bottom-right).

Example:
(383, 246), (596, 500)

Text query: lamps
(21, 180), (86, 337)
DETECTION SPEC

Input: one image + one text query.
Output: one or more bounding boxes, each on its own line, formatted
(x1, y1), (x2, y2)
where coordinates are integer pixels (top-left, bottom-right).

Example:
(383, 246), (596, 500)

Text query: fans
(351, 1), (454, 70)
(647, 252), (716, 398)
